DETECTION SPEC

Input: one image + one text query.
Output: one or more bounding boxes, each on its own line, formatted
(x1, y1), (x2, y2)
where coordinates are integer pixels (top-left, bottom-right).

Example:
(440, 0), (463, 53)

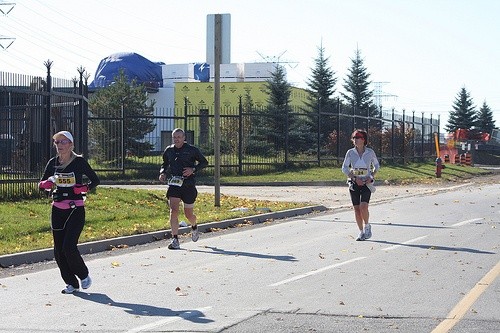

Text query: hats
(53, 131), (74, 143)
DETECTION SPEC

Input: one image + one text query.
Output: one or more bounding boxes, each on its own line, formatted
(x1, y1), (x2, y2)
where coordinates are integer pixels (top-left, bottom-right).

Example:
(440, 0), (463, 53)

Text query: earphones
(70, 145), (71, 148)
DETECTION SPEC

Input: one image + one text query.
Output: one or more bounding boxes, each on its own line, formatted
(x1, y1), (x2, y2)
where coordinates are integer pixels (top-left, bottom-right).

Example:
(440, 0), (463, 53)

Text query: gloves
(40, 180), (56, 189)
(74, 184), (90, 194)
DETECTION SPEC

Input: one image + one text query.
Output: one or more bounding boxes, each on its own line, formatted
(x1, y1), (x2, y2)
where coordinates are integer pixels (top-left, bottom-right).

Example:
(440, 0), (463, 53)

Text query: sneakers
(168, 238), (180, 249)
(62, 285), (79, 294)
(81, 275), (92, 289)
(191, 225), (199, 242)
(356, 233), (365, 241)
(364, 224), (372, 239)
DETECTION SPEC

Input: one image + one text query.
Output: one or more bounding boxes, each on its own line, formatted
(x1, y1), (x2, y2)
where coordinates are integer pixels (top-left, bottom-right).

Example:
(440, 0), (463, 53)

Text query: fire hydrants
(435, 157), (447, 179)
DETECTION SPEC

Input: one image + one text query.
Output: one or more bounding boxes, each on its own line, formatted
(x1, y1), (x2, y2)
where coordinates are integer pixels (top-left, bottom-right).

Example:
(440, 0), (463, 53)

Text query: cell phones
(69, 201), (78, 214)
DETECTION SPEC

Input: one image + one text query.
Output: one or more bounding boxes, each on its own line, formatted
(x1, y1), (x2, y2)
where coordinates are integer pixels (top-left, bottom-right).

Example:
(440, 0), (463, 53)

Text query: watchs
(192, 167), (196, 173)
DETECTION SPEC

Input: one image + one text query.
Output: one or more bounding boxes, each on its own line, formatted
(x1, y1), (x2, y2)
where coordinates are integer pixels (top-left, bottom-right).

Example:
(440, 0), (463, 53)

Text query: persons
(38, 131), (100, 294)
(341, 130), (380, 241)
(159, 128), (209, 249)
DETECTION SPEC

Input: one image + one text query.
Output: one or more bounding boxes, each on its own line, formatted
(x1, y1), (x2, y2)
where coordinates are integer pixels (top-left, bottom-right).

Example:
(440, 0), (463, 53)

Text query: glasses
(54, 140), (72, 145)
(352, 136), (363, 139)
